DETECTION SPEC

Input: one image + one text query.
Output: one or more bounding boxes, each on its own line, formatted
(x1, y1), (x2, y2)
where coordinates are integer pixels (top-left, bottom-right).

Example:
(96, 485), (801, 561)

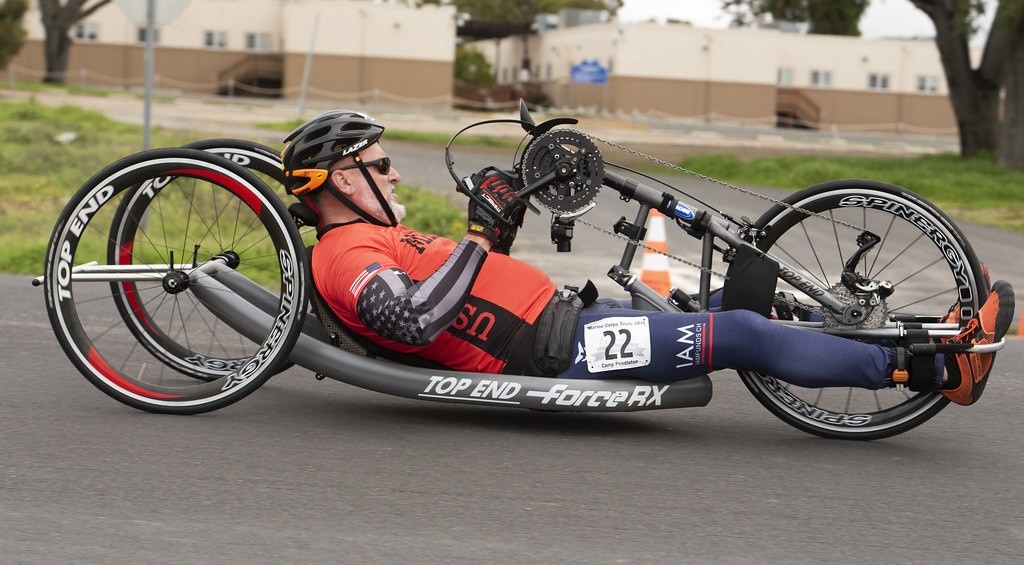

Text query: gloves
(467, 175), (524, 247)
(491, 194), (530, 256)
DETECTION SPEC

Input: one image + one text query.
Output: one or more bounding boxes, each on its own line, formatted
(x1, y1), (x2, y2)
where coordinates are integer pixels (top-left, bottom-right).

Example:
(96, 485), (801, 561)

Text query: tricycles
(31, 95), (1007, 442)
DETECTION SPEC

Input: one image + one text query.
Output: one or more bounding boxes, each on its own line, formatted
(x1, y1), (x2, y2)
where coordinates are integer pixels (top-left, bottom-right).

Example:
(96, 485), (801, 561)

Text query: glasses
(329, 157), (391, 178)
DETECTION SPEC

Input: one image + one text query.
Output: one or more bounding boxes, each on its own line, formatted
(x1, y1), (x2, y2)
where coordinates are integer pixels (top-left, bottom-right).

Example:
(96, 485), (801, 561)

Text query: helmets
(280, 110), (386, 196)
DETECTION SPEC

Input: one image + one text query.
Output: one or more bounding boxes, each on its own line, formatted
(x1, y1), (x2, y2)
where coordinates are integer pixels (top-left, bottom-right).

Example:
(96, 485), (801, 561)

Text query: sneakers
(938, 279), (1015, 406)
(932, 259), (990, 345)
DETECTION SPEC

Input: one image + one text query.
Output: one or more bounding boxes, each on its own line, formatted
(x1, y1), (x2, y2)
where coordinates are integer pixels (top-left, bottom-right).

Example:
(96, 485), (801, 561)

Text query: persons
(281, 111), (1015, 406)
